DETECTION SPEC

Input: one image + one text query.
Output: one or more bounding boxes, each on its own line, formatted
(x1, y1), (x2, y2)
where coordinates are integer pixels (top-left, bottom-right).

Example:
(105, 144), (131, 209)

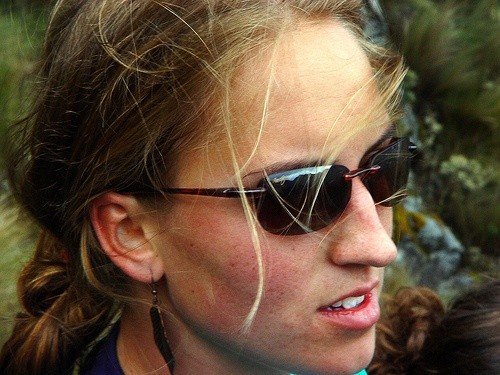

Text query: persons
(366, 274), (500, 375)
(0, 0), (414, 375)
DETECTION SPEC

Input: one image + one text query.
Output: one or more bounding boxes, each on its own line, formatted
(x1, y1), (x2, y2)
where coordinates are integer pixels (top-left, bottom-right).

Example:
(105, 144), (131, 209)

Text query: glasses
(121, 136), (418, 236)
